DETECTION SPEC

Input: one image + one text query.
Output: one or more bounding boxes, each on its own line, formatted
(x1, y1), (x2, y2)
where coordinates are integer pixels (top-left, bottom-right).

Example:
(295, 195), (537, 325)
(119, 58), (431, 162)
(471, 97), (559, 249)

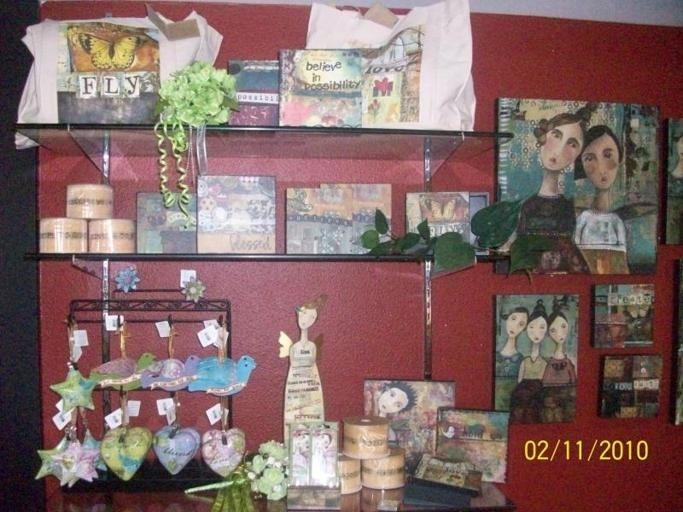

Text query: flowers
(153, 63), (242, 125)
(244, 440), (288, 500)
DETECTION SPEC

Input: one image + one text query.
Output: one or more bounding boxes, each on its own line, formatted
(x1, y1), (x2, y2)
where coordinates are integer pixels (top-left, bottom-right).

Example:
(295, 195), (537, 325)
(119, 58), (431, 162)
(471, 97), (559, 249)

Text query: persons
(293, 433), (312, 486)
(312, 433), (332, 483)
(375, 382), (417, 447)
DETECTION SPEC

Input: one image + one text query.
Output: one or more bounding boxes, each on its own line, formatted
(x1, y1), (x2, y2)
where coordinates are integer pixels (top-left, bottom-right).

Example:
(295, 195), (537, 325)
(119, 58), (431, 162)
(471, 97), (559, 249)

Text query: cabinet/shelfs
(14, 123), (516, 512)
